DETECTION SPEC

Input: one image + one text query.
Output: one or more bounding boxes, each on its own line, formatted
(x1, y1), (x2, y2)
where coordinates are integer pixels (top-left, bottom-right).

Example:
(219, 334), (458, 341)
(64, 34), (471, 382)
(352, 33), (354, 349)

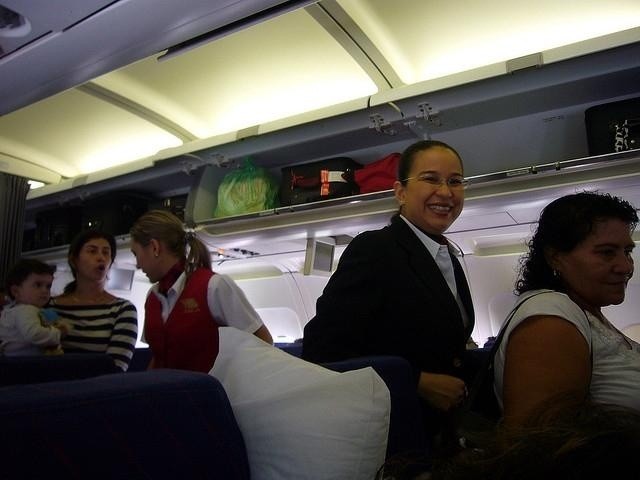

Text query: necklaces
(70, 294), (104, 304)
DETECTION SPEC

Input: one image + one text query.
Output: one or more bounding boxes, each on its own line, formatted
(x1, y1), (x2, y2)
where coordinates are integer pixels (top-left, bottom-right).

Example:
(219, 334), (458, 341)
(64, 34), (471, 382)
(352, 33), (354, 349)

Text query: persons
(130, 209), (274, 376)
(489, 190), (640, 449)
(300, 138), (478, 457)
(43, 223), (139, 374)
(2, 257), (71, 357)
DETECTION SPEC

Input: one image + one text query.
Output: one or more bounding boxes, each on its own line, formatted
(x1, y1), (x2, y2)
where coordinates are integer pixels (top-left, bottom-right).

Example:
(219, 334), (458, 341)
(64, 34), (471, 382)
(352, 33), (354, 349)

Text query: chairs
(0, 337), (495, 480)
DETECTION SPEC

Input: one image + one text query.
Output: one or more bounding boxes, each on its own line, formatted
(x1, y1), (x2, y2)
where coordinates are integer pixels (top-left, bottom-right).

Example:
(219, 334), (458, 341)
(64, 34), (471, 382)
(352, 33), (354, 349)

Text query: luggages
(279, 157), (364, 206)
(32, 191), (188, 250)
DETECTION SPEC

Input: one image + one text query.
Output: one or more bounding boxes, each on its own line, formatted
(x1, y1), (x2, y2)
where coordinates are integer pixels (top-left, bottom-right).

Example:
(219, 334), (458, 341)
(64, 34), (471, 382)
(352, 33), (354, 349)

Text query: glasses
(403, 173), (472, 192)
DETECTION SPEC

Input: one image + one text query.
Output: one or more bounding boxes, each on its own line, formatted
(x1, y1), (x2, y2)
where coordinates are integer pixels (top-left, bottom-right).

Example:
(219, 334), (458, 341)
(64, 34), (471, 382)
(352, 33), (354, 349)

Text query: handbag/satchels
(584, 96), (640, 155)
(353, 153), (401, 193)
